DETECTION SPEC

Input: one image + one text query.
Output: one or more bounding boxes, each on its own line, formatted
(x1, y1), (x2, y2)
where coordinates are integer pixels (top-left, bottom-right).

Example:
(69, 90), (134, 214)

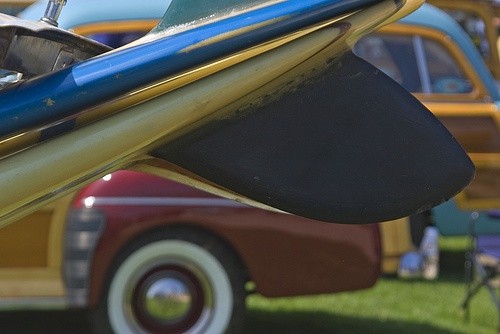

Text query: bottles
(421, 225), (440, 280)
(399, 249), (423, 278)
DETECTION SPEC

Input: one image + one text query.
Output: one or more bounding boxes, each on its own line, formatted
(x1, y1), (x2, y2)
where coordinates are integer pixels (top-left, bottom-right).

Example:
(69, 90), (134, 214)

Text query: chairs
(459, 210), (500, 325)
